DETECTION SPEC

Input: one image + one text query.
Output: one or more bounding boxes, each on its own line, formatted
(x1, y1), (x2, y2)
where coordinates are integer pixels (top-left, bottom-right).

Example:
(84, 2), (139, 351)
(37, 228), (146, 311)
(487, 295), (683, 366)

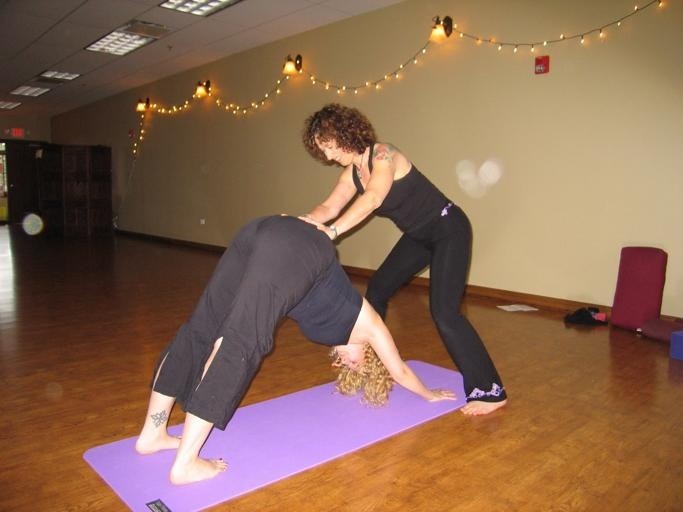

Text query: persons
(280, 102), (509, 416)
(134, 214), (459, 485)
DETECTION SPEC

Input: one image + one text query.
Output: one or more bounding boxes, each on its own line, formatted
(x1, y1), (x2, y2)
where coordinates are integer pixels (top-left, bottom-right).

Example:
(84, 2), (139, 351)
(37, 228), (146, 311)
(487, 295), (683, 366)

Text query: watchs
(330, 225), (340, 238)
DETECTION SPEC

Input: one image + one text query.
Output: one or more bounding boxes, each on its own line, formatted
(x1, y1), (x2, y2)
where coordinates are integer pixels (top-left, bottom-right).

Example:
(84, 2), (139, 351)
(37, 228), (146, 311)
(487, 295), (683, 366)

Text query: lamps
(136, 97), (149, 112)
(196, 80), (210, 97)
(283, 54), (302, 74)
(429, 15), (452, 43)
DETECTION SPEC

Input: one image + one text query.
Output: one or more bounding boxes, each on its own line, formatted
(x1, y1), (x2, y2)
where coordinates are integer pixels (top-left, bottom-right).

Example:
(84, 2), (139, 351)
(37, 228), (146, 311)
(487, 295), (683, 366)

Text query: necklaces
(354, 153), (365, 182)
